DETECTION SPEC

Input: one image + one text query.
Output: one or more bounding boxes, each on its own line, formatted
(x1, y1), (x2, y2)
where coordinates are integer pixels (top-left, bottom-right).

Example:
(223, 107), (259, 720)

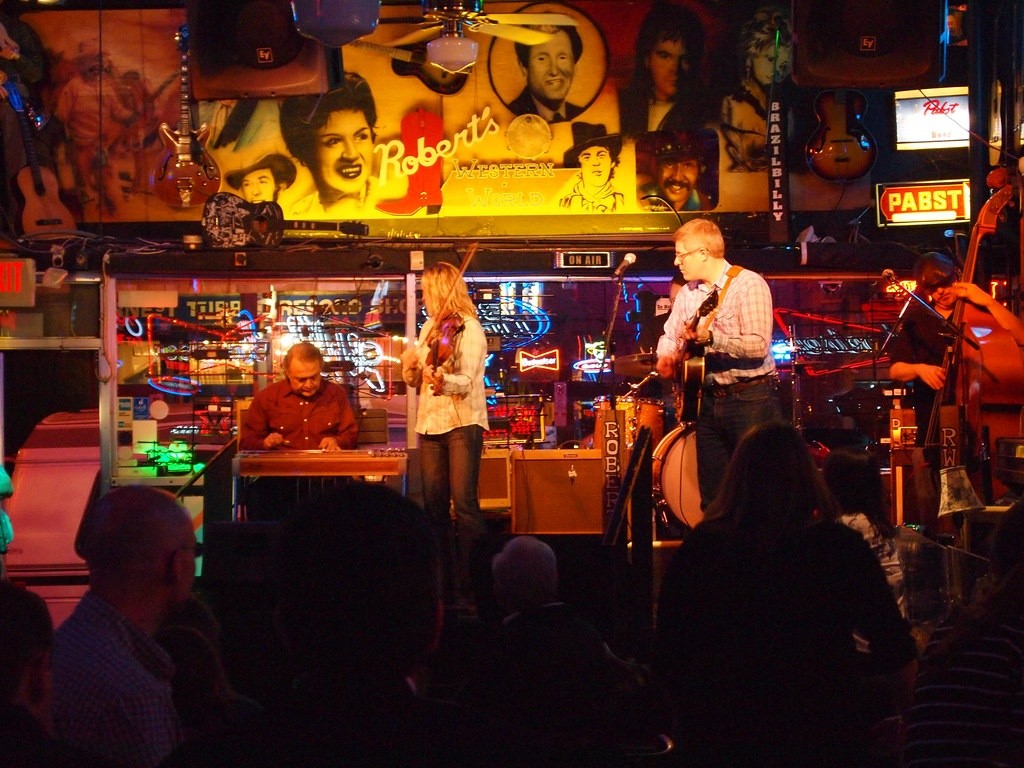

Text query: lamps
(937, 465), (985, 553)
(426, 21), (479, 75)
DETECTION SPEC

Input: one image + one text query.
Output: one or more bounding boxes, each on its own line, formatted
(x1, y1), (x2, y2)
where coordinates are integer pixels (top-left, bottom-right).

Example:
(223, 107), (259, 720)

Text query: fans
(378, 0), (580, 48)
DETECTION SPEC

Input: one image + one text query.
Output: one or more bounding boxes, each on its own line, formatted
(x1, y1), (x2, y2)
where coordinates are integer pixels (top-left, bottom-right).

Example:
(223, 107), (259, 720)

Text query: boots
(374, 105), (444, 217)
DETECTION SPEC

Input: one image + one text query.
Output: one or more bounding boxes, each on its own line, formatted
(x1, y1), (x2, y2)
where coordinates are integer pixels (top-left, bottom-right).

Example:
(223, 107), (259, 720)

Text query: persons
(651, 418), (920, 768)
(53, 48), (157, 219)
(26, 486), (261, 768)
(240, 343), (358, 522)
(901, 496), (1024, 768)
(640, 131), (712, 211)
(151, 479), (556, 768)
(504, 24), (584, 123)
(279, 71), (381, 219)
(889, 251), (1024, 448)
(620, 1), (706, 138)
(471, 537), (625, 725)
(224, 152), (297, 204)
(400, 262), (489, 522)
(0, 580), (59, 768)
(631, 219), (784, 455)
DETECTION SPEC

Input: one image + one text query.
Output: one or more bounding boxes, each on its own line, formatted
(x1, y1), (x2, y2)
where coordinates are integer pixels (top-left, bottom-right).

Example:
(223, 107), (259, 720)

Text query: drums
(651, 421), (704, 539)
(593, 395), (664, 469)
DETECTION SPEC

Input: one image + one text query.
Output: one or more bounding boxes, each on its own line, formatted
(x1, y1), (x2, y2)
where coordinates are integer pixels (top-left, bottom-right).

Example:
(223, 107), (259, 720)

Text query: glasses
(674, 249), (698, 258)
(922, 277), (953, 294)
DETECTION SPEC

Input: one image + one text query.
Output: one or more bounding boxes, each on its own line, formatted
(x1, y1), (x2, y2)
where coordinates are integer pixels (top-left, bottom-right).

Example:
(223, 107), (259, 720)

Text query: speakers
(187, 0), (329, 101)
(477, 448), (515, 509)
(789, 0), (947, 92)
(509, 448), (626, 535)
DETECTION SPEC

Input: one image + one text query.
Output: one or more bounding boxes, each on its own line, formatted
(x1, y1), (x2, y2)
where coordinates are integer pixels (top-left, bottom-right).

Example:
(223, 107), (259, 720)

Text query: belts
(704, 375), (772, 398)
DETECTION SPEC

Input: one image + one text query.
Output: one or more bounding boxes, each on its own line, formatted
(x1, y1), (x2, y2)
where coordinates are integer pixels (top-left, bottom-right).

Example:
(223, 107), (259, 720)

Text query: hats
(650, 127), (707, 163)
(223, 142), (298, 190)
(562, 122), (624, 166)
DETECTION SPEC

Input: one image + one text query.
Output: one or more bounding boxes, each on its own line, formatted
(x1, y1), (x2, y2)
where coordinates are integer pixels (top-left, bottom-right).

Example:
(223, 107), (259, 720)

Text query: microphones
(613, 253), (636, 277)
(882, 269), (895, 279)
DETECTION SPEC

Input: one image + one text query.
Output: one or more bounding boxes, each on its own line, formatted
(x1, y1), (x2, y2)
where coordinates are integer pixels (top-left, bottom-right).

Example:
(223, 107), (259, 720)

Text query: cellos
(924, 167), (1024, 538)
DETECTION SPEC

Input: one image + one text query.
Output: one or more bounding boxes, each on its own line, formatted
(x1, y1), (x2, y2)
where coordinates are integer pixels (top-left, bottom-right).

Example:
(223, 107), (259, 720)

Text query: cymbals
(776, 360), (828, 367)
(608, 353), (658, 379)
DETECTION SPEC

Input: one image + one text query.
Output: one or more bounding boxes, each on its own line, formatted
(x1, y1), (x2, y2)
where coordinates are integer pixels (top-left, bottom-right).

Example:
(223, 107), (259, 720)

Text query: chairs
(235, 408), (388, 452)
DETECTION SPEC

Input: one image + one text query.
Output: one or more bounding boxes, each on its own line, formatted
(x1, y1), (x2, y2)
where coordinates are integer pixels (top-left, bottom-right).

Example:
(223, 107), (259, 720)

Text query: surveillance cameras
(367, 255), (383, 269)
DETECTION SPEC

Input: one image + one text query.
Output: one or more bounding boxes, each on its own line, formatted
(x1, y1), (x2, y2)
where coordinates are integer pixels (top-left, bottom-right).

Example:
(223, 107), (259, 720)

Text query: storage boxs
(478, 449), (606, 535)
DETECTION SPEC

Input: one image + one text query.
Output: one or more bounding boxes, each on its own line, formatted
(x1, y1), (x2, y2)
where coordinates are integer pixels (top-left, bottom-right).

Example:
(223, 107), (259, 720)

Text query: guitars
(672, 285), (725, 424)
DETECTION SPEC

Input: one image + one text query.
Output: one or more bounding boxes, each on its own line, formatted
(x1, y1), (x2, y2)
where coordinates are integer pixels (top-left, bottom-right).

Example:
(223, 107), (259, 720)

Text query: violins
(425, 311), (465, 396)
